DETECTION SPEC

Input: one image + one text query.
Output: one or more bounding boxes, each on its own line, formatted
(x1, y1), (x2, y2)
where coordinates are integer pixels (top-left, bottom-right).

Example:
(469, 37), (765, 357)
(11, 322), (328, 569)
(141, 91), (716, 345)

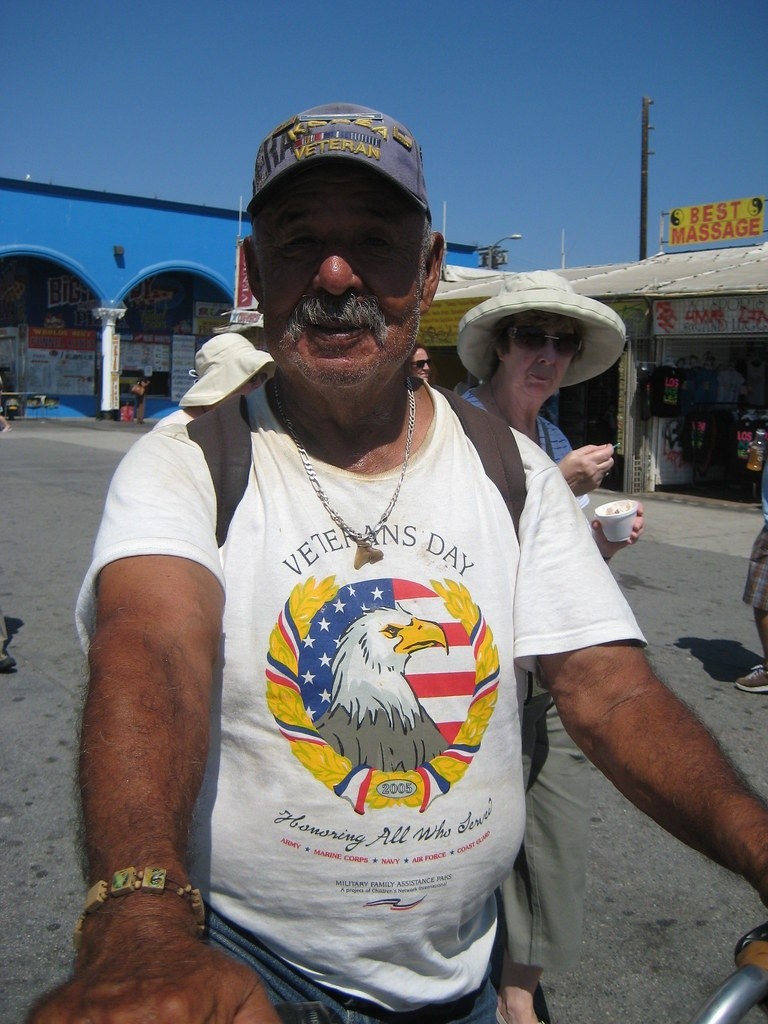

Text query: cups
(593, 499), (640, 542)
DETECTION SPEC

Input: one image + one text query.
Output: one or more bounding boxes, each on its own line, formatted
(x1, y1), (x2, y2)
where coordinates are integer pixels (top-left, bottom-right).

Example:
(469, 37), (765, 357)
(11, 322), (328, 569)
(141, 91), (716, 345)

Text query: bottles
(747, 429), (766, 470)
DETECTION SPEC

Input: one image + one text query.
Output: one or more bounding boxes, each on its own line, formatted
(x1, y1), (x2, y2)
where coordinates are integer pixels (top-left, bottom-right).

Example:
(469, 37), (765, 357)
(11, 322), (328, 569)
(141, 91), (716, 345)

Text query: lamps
(114, 245), (123, 258)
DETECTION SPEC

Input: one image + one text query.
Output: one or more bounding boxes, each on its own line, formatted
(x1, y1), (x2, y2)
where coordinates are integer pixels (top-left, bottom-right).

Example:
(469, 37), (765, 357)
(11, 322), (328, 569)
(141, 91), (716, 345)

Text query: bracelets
(73, 867), (205, 949)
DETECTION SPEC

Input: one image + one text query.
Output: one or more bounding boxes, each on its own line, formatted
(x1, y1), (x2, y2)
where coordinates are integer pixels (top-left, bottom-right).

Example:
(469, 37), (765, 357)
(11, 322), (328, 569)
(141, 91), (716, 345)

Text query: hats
(245, 100), (433, 232)
(457, 274), (629, 384)
(179, 332), (275, 404)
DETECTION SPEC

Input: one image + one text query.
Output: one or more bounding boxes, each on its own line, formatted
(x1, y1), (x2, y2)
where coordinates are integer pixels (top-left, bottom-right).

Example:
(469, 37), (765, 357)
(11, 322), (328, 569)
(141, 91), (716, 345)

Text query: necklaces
(273, 377), (416, 570)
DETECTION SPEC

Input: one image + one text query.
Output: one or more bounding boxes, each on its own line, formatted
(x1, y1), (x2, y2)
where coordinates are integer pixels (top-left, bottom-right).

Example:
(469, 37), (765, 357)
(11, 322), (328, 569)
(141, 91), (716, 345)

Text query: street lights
(489, 234), (522, 271)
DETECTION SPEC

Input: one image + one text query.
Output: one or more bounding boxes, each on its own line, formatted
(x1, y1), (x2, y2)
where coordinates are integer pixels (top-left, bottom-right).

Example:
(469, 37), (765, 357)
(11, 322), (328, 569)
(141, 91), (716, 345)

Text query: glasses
(498, 323), (588, 357)
(249, 371), (267, 384)
(410, 358), (435, 369)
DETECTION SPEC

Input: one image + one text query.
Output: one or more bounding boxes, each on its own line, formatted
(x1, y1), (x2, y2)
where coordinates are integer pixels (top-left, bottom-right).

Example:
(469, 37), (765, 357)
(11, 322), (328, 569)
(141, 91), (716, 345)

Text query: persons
(0, 416), (17, 671)
(457, 270), (645, 1024)
(152, 333), (767, 693)
(26, 103), (768, 1024)
(136, 376), (150, 424)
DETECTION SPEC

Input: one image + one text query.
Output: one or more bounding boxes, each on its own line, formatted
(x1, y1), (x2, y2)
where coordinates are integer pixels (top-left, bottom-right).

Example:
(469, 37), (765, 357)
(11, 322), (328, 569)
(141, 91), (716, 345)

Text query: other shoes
(2, 424), (14, 433)
(0, 650), (17, 671)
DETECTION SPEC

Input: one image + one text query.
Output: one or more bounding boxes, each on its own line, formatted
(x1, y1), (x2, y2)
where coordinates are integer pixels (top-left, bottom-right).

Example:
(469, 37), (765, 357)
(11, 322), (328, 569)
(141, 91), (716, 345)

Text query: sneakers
(736, 661), (767, 695)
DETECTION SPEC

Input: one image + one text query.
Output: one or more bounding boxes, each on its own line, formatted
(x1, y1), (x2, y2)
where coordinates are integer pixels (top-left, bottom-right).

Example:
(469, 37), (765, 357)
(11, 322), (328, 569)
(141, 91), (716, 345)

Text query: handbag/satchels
(133, 385), (145, 394)
